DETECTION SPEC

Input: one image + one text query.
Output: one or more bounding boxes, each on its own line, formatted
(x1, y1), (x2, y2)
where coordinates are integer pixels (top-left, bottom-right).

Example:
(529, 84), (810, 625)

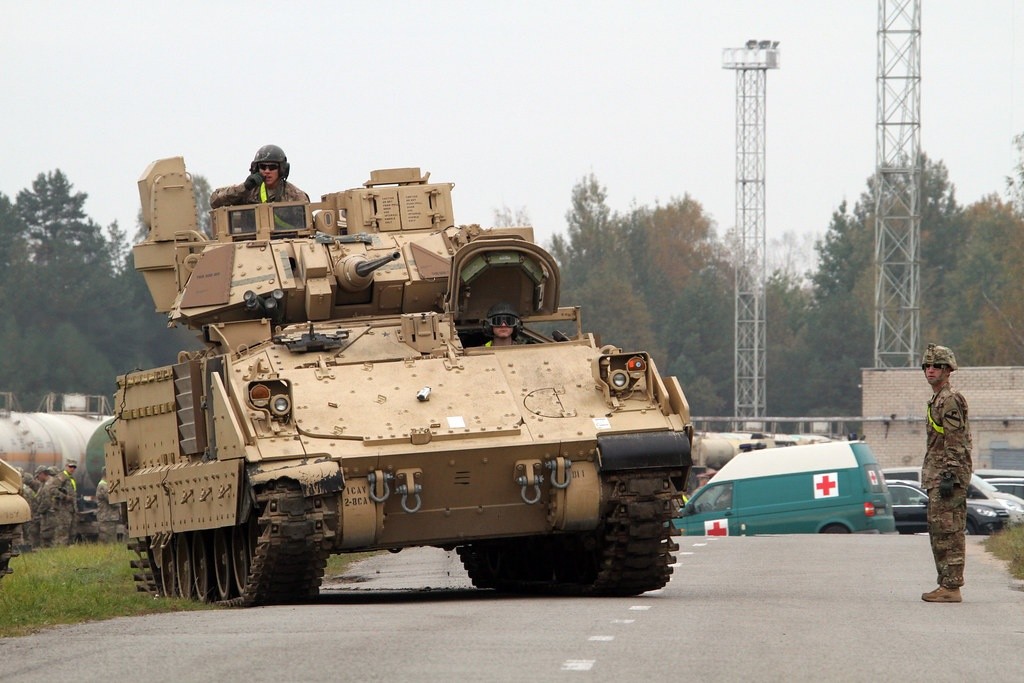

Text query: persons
(920, 342), (973, 603)
(482, 301), (529, 348)
(210, 145), (310, 234)
(18, 458), (122, 548)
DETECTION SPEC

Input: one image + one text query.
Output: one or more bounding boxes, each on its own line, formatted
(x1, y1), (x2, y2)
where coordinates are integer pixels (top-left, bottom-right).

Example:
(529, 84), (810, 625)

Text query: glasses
(924, 363), (942, 369)
(258, 163), (278, 171)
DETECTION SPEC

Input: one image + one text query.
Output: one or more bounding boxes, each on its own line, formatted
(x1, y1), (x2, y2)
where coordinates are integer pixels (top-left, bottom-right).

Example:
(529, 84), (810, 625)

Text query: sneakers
(921, 587), (962, 602)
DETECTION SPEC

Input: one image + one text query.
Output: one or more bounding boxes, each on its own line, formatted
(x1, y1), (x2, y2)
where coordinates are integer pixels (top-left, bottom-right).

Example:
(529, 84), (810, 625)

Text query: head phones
(482, 302), (523, 338)
(251, 145), (290, 179)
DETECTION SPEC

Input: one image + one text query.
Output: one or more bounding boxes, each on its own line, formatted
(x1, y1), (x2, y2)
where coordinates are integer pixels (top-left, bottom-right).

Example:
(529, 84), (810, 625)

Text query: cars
(886, 480), (1010, 536)
(881, 468), (1024, 524)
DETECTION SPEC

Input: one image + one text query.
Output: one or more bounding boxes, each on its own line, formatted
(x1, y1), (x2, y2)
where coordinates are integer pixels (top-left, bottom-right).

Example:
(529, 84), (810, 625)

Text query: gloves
(243, 173), (262, 191)
(939, 478), (954, 500)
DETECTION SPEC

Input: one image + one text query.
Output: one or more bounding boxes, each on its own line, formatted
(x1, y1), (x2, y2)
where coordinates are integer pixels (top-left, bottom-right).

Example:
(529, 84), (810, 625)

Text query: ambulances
(664, 440), (899, 537)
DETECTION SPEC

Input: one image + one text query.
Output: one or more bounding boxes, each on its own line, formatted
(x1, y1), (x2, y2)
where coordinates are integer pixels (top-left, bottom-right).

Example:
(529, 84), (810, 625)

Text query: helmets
(921, 343), (958, 371)
(248, 144), (290, 180)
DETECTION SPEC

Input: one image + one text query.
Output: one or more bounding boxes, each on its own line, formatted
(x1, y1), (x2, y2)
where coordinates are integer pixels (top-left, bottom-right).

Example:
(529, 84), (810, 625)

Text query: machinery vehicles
(0, 459), (33, 580)
(105, 155), (694, 608)
